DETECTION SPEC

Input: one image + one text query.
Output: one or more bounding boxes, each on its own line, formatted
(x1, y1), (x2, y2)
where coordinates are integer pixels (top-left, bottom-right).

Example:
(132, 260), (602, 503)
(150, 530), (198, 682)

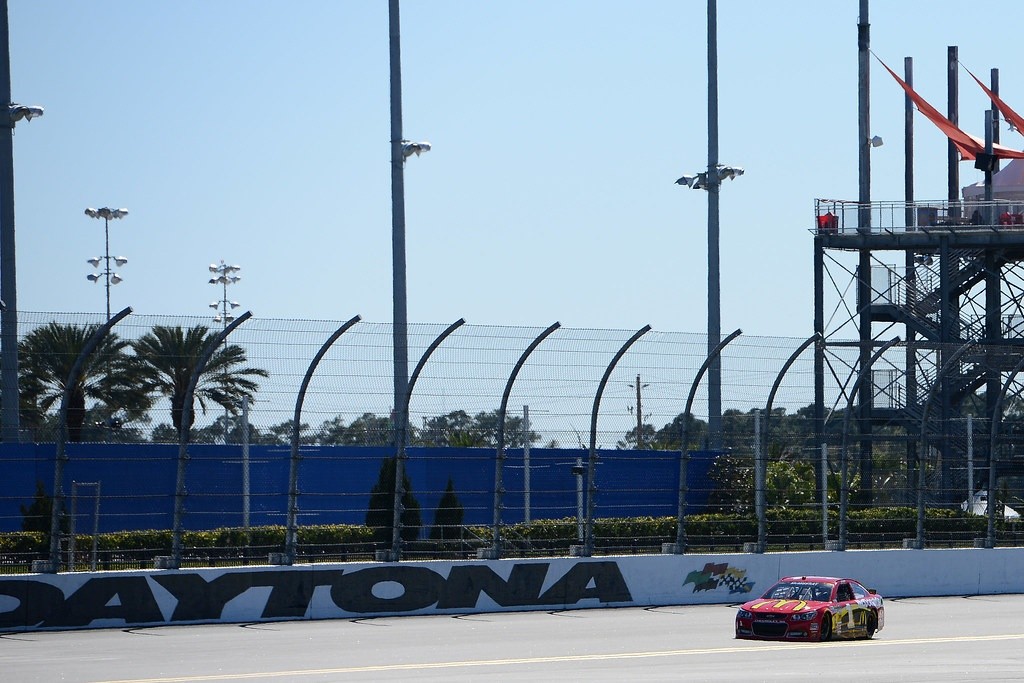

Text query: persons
(969, 210), (985, 225)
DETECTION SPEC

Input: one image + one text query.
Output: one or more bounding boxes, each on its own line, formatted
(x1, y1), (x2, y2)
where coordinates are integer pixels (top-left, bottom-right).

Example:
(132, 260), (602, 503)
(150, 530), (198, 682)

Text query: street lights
(627, 374), (653, 451)
(84, 204), (131, 448)
(391, 140), (428, 447)
(0, 100), (43, 444)
(207, 257), (242, 446)
(676, 166), (747, 451)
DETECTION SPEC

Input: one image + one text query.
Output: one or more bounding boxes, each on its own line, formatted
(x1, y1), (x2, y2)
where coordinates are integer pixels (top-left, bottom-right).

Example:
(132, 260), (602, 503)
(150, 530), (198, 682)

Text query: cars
(736, 575), (882, 642)
